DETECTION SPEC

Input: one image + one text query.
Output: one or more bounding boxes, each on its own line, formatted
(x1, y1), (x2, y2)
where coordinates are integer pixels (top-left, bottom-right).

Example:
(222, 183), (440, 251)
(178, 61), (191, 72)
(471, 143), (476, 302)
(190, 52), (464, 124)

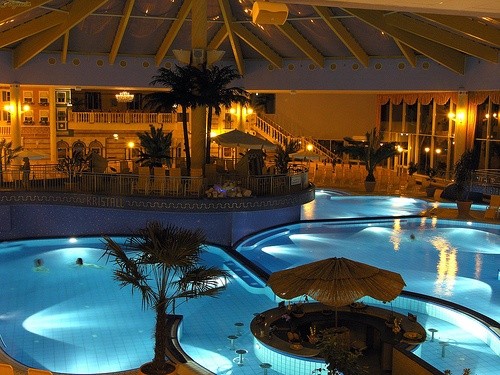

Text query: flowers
(293, 303), (304, 311)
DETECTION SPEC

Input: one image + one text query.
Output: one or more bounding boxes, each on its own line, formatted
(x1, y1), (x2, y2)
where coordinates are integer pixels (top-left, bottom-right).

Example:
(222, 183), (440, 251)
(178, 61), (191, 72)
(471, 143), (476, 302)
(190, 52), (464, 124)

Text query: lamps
(115, 90), (134, 102)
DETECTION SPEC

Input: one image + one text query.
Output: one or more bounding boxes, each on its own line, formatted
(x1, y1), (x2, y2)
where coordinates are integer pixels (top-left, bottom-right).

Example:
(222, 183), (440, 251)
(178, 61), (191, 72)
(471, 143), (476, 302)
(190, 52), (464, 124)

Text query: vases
(292, 311), (305, 318)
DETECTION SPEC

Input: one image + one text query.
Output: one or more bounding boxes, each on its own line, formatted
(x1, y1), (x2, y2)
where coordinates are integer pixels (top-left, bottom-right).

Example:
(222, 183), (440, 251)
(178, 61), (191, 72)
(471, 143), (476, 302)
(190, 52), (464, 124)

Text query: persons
(20, 157), (30, 189)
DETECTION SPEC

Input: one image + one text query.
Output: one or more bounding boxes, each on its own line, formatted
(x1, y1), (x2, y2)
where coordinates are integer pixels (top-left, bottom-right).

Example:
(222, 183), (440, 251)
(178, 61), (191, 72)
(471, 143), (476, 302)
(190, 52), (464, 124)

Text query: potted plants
(100, 220), (234, 375)
(455, 149), (478, 218)
(407, 161), (421, 186)
(340, 127), (397, 192)
(426, 168), (438, 197)
(64, 150), (94, 189)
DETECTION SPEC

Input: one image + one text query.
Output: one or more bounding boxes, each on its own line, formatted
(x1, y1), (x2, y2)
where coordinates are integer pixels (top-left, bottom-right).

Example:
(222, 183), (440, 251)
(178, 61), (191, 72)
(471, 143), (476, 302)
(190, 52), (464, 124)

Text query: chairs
(119, 155), (275, 198)
(485, 195), (500, 218)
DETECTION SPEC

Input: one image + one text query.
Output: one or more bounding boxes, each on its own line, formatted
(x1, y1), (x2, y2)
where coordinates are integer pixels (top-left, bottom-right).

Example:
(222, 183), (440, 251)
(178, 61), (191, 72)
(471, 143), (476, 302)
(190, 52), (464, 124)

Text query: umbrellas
(3, 149), (51, 160)
(269, 256), (405, 328)
(211, 128), (274, 169)
